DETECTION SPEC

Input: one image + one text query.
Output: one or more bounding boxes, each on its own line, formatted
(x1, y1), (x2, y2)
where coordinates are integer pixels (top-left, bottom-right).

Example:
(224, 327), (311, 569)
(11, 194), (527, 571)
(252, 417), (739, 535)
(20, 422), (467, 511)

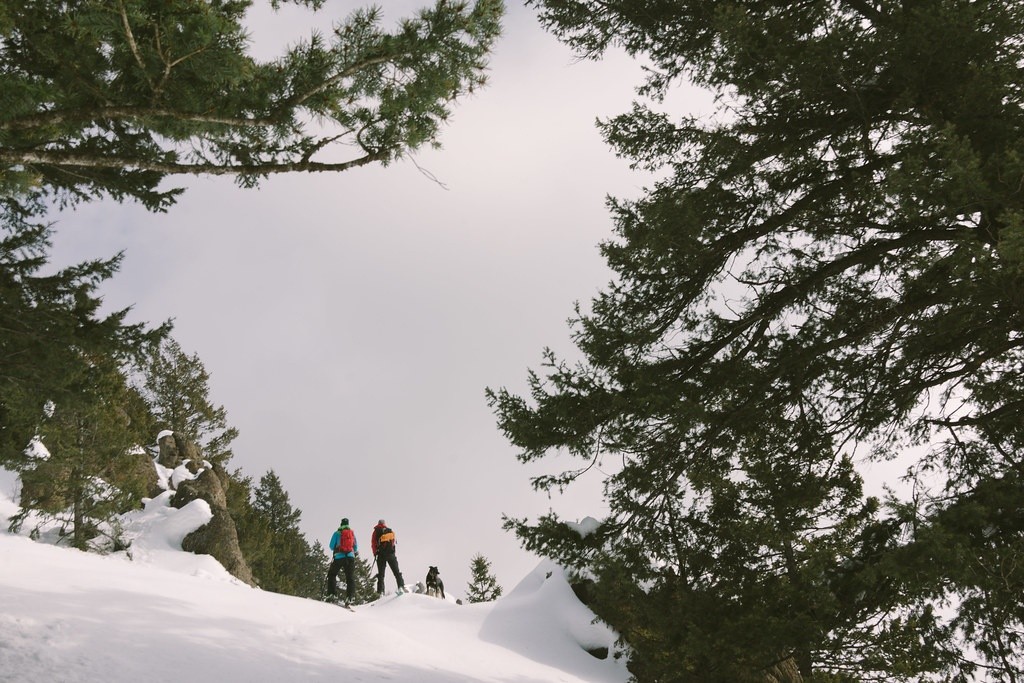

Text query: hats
(378, 519), (385, 526)
(340, 518), (349, 525)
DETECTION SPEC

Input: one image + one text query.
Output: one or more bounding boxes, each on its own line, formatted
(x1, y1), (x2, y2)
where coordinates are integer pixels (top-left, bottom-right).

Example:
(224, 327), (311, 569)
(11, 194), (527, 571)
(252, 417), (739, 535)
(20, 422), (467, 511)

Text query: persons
(324, 518), (359, 606)
(371, 520), (405, 598)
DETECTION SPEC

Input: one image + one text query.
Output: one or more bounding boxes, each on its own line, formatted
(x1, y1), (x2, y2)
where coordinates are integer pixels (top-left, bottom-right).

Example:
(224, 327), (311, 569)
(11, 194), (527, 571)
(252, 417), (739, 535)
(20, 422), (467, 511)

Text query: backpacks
(337, 529), (354, 553)
(376, 527), (394, 553)
